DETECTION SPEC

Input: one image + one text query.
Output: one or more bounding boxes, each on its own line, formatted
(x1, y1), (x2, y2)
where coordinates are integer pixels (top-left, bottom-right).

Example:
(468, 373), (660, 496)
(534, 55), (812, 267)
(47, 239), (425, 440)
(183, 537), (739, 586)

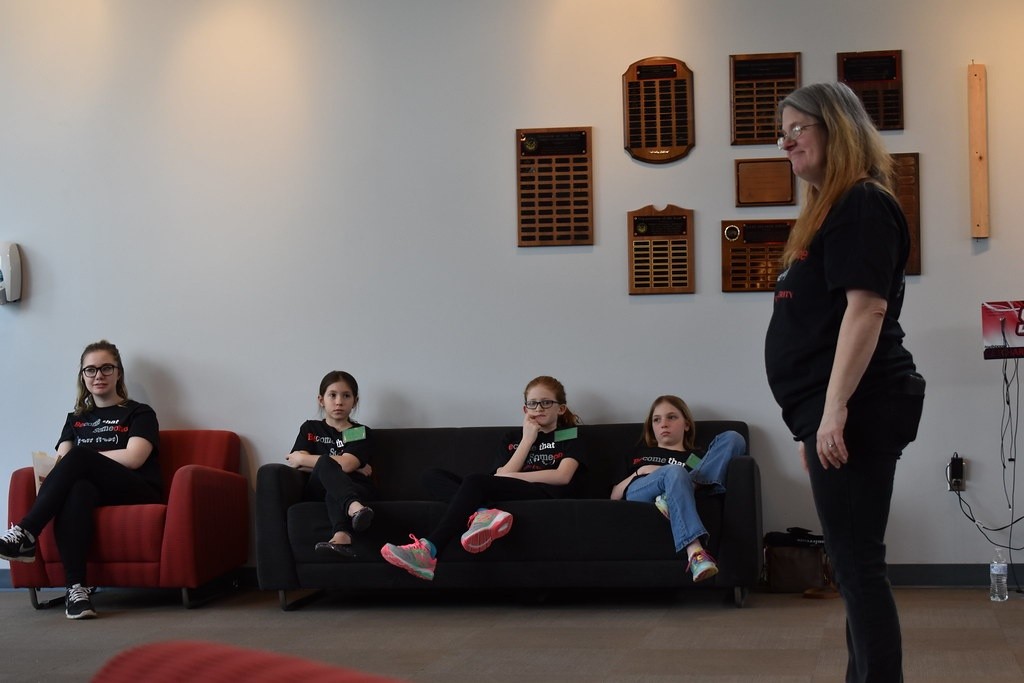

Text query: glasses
(82, 364), (119, 378)
(778, 122), (822, 150)
(525, 400), (559, 409)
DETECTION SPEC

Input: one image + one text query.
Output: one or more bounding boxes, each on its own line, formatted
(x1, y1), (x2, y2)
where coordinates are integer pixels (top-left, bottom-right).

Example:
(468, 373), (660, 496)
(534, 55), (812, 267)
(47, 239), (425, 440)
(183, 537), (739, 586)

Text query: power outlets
(947, 463), (966, 490)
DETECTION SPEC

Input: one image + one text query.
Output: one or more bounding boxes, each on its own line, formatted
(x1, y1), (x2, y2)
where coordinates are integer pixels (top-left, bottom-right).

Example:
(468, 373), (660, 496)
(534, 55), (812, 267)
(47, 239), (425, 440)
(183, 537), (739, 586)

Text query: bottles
(989, 546), (1009, 601)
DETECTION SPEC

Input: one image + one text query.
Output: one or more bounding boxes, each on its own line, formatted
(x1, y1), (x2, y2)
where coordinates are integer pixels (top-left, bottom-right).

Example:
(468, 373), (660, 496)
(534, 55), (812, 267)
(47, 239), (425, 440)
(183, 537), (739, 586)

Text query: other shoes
(314, 542), (351, 559)
(352, 507), (374, 532)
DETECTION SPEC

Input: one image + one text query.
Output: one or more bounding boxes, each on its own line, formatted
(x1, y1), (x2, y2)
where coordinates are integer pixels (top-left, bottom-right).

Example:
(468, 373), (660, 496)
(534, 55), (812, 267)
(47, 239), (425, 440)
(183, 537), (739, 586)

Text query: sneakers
(65, 583), (97, 619)
(461, 509), (513, 553)
(655, 493), (670, 521)
(686, 549), (719, 582)
(0, 522), (36, 563)
(380, 534), (436, 581)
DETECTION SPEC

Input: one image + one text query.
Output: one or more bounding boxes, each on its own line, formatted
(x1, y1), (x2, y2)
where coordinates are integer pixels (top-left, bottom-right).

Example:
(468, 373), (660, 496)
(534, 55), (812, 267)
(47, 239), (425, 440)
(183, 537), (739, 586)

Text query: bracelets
(636, 470), (638, 476)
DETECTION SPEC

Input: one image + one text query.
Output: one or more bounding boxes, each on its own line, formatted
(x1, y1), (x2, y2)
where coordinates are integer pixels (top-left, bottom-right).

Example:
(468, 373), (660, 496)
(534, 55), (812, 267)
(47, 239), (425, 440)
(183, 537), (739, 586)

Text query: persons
(287, 371), (380, 558)
(0, 341), (161, 618)
(381, 376), (582, 581)
(611, 395), (745, 582)
(765, 82), (924, 683)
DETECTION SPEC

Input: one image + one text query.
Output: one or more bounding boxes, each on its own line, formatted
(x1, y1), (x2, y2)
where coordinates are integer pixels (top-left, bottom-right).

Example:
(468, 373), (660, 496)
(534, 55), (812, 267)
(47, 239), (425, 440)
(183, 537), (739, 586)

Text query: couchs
(7, 428), (252, 611)
(252, 419), (763, 607)
